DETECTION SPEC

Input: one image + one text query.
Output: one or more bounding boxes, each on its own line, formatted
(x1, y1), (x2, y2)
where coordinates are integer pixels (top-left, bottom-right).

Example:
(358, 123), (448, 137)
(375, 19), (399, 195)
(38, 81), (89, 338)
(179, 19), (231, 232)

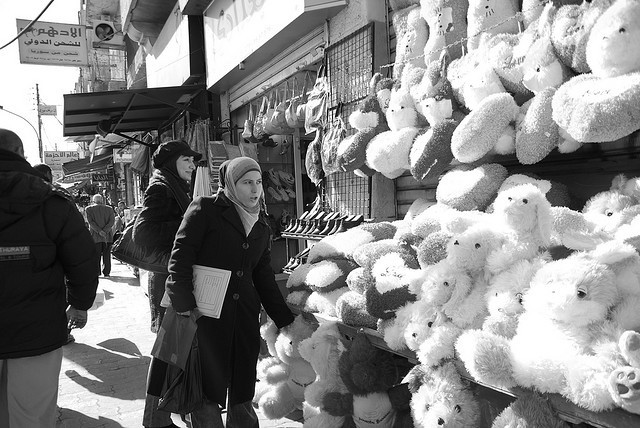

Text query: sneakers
(278, 169), (294, 187)
(276, 186), (290, 201)
(267, 168), (282, 187)
(284, 186), (296, 199)
(267, 186), (282, 201)
(279, 140), (289, 155)
(262, 138), (278, 149)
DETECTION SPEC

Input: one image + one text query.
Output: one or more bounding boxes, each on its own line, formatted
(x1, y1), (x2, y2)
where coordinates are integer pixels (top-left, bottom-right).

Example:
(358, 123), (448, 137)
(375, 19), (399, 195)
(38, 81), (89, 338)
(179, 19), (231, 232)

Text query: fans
(94, 21), (116, 41)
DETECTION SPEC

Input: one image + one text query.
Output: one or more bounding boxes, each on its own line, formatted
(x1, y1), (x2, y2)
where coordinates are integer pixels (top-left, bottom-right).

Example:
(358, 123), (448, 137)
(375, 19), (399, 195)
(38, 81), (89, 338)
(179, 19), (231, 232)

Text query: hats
(151, 140), (203, 168)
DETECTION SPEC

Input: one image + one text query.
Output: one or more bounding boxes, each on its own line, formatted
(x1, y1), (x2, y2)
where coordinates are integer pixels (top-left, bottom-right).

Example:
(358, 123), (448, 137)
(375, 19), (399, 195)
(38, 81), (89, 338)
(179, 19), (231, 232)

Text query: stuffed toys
(522, 0), (579, 29)
(552, 2), (640, 143)
(467, 0), (522, 50)
(514, 2), (584, 166)
(255, 163), (640, 427)
(255, 166), (640, 428)
(336, 72), (394, 177)
(447, 31), (520, 163)
(364, 63), (425, 179)
(410, 60), (466, 181)
(391, 6), (427, 80)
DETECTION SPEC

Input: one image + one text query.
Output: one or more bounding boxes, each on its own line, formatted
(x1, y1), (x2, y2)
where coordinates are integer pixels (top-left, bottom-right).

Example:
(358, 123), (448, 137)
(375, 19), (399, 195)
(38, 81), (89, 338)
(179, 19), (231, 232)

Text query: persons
(165, 156), (294, 428)
(114, 201), (129, 231)
(1, 127), (99, 428)
(143, 140), (203, 428)
(33, 164), (74, 201)
(86, 194), (114, 279)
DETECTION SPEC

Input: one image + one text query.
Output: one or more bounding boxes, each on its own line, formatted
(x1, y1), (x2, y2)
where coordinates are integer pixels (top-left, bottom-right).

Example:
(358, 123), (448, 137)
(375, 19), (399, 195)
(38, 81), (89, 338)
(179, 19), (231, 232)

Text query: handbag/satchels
(285, 77), (304, 127)
(241, 103), (262, 143)
(295, 70), (314, 121)
(109, 182), (183, 274)
(253, 96), (267, 140)
(304, 64), (329, 136)
(320, 101), (348, 178)
(156, 330), (204, 415)
(150, 308), (204, 370)
(262, 91), (275, 136)
(305, 126), (325, 187)
(270, 81), (295, 134)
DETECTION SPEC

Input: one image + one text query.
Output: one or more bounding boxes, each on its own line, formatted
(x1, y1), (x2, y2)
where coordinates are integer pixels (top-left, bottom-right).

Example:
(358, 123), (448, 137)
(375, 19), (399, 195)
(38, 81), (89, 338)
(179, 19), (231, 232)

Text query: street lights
(0, 106), (44, 163)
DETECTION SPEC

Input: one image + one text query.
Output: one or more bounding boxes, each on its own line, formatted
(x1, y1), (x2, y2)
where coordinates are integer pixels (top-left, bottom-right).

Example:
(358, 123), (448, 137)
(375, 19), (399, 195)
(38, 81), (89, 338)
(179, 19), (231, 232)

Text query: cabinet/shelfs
(277, 213), (363, 279)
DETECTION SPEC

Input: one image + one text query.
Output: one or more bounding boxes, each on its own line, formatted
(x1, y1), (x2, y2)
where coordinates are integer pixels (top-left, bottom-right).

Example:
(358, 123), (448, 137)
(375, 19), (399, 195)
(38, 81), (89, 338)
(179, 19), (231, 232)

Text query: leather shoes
(283, 209), (363, 238)
(282, 248), (312, 272)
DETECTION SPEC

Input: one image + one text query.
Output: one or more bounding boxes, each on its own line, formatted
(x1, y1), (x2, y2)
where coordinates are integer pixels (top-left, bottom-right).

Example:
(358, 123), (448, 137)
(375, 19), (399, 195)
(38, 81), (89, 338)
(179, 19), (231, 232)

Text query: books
(420, 0), (469, 63)
